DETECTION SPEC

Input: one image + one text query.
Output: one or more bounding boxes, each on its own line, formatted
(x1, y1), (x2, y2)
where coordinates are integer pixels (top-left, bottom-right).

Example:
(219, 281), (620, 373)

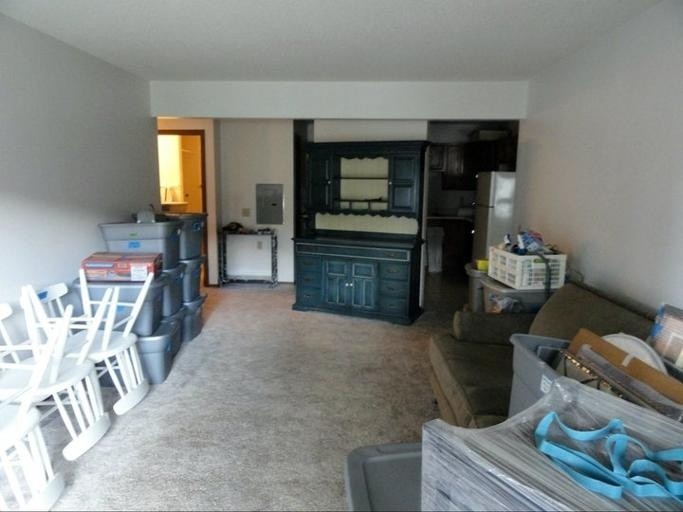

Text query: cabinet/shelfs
(428, 218), (474, 266)
(441, 141), (520, 192)
(292, 138), (428, 327)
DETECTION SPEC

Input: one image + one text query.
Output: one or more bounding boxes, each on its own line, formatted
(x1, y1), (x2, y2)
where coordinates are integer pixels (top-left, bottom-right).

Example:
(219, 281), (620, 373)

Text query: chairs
(1, 316), (67, 512)
(22, 268), (154, 417)
(1, 281), (113, 462)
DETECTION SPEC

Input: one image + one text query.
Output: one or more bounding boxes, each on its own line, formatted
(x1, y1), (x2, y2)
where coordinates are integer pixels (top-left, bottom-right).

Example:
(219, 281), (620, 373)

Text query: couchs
(411, 282), (682, 436)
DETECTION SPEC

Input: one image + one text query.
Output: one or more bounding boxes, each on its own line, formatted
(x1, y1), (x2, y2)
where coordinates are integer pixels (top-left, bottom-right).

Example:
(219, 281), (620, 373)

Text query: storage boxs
(344, 440), (423, 511)
(508, 332), (683, 423)
(642, 304), (683, 371)
(462, 261), (491, 306)
(478, 277), (555, 315)
(72, 211), (209, 386)
